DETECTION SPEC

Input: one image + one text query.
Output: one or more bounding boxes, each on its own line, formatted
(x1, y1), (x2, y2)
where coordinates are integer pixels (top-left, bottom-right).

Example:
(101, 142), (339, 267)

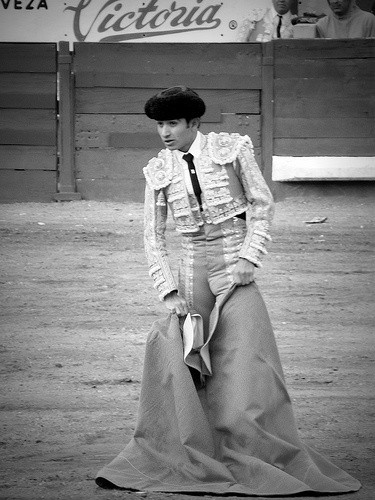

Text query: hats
(144, 85), (206, 121)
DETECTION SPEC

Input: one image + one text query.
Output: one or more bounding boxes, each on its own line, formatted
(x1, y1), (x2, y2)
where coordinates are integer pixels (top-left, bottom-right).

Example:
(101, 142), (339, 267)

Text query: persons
(95, 85), (361, 496)
(243, 0), (300, 44)
(316, 0), (375, 39)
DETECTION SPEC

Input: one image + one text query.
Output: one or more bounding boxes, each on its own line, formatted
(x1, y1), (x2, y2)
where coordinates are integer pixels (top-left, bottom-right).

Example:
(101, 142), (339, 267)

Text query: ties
(276, 13), (282, 39)
(182, 153), (203, 213)
